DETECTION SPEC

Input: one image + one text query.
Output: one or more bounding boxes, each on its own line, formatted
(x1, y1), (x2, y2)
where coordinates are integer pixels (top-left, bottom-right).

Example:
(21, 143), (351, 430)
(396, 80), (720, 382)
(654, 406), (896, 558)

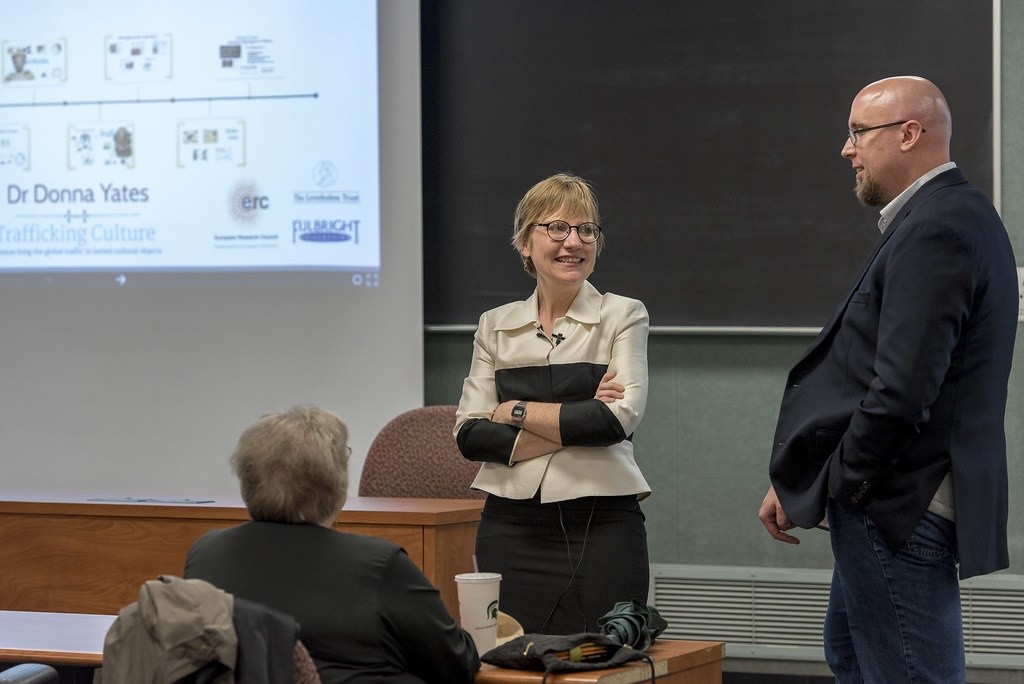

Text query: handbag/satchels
(481, 633), (646, 673)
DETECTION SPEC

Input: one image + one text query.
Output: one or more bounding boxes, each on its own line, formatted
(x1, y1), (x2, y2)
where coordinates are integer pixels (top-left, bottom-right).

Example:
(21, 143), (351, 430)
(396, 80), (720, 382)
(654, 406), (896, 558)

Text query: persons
(755, 75), (1023, 684)
(453, 169), (652, 635)
(183, 399), (482, 684)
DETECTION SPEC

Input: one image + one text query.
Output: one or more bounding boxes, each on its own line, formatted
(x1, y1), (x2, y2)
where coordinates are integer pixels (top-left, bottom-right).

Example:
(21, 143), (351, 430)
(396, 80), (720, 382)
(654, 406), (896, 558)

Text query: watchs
(510, 399), (528, 429)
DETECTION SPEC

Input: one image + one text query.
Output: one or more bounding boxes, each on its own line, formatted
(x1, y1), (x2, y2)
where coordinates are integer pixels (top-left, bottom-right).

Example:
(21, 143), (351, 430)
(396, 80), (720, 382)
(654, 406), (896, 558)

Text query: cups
(454, 572), (502, 658)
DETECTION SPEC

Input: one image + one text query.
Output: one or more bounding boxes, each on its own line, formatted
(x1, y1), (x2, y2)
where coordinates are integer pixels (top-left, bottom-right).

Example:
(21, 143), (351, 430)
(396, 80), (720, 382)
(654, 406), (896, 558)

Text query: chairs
(358, 404), (496, 501)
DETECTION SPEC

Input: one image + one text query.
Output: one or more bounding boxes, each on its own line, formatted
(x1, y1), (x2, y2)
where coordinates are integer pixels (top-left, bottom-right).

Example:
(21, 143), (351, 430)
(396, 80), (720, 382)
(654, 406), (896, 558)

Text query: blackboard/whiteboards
(419, 2), (1004, 334)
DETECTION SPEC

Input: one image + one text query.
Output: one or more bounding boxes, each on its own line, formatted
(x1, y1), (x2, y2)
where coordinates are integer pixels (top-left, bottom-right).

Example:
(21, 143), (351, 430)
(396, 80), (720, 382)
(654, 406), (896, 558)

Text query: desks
(0, 491), (727, 683)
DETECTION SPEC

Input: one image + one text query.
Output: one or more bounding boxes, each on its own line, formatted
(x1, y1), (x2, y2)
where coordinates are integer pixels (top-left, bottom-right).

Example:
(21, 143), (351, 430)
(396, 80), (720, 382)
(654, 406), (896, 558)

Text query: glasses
(528, 219), (601, 244)
(848, 120), (925, 145)
(345, 445), (352, 462)
